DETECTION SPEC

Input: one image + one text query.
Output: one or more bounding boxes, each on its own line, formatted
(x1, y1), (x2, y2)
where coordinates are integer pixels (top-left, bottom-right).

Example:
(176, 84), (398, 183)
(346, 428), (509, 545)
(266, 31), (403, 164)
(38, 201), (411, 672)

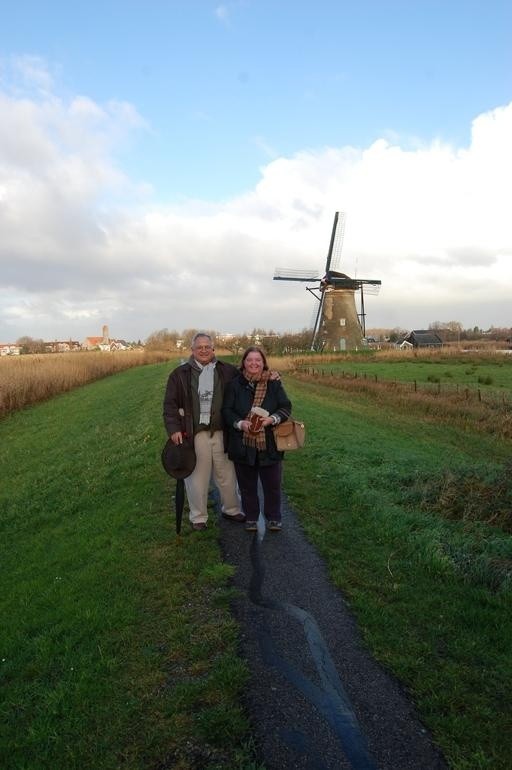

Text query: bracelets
(270, 415), (277, 426)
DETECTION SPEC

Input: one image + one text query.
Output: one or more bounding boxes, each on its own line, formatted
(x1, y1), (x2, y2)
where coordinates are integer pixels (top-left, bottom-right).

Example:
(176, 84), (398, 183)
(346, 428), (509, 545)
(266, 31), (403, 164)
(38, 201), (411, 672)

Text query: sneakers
(245, 520), (258, 530)
(266, 520), (282, 530)
(193, 522), (208, 532)
(222, 511), (248, 524)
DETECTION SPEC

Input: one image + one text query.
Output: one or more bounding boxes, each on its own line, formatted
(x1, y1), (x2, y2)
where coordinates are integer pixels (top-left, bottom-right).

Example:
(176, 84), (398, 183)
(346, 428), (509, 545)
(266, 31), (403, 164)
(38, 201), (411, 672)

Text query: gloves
(248, 406), (269, 433)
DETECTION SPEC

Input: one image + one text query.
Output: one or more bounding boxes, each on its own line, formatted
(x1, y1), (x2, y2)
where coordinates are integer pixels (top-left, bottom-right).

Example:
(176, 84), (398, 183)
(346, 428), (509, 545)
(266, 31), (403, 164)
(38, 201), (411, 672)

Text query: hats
(161, 438), (197, 480)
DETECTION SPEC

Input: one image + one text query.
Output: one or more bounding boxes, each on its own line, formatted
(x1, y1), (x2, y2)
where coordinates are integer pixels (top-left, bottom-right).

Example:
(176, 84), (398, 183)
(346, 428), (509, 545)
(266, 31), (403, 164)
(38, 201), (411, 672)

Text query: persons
(222, 346), (292, 532)
(163, 333), (246, 532)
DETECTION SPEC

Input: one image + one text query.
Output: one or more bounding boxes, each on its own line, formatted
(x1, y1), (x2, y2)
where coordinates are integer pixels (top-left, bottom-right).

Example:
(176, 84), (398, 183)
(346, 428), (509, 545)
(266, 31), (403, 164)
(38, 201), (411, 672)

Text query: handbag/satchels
(272, 420), (306, 452)
